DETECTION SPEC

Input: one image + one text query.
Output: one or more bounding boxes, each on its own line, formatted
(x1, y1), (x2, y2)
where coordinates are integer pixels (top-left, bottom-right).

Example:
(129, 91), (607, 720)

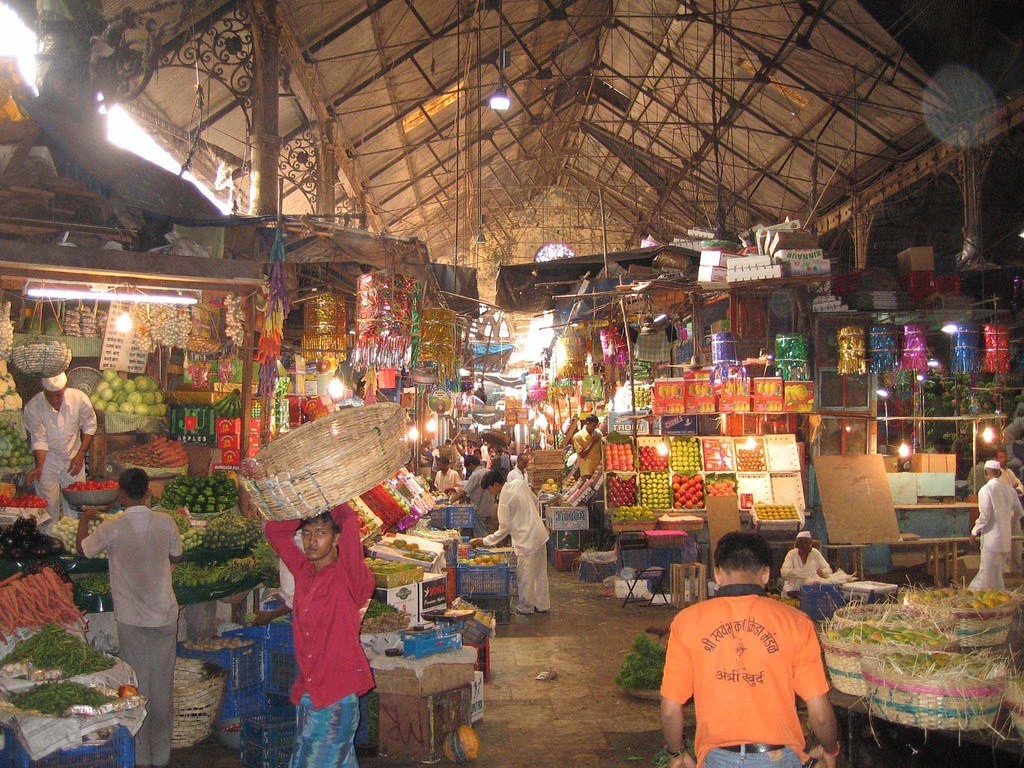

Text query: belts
(718, 740), (784, 755)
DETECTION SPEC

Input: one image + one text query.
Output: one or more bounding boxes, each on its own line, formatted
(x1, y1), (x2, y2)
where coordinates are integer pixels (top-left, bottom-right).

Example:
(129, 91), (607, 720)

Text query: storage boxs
(697, 266), (727, 282)
(764, 228), (808, 254)
(910, 453), (959, 475)
(896, 246), (935, 271)
(727, 254), (771, 269)
(799, 584), (843, 618)
(884, 456), (898, 472)
(774, 249), (823, 260)
(726, 264), (782, 283)
(908, 289), (933, 308)
(700, 250), (743, 267)
(784, 258), (831, 277)
(687, 229), (715, 239)
(842, 581), (898, 604)
(917, 473), (956, 497)
(169, 352), (816, 768)
(936, 275), (962, 297)
(0, 724), (13, 768)
(757, 219), (801, 253)
(769, 232), (820, 256)
(14, 726), (136, 768)
(899, 272), (934, 289)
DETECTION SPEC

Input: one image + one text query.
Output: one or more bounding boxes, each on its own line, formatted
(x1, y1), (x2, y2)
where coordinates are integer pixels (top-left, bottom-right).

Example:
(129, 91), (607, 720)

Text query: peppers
(361, 599), (401, 619)
(77, 543), (279, 596)
(0, 621), (117, 719)
(150, 472), (239, 513)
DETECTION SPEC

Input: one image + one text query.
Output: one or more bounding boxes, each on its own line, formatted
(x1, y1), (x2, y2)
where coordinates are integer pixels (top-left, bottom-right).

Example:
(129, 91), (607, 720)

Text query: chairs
(618, 531), (669, 614)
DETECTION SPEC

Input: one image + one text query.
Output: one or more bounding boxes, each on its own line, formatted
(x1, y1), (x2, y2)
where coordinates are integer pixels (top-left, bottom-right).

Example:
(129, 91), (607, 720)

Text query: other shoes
(534, 605), (548, 614)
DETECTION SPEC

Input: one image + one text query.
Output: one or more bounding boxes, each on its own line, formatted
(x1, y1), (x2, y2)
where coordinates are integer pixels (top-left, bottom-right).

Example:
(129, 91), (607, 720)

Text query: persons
(24, 372), (97, 524)
(507, 454), (530, 483)
(419, 439), (533, 471)
(967, 449), (1024, 590)
(450, 455), (494, 539)
(434, 457), (462, 505)
(266, 502), (376, 768)
(573, 414), (603, 481)
(660, 531), (839, 768)
(76, 468), (183, 768)
(469, 471), (553, 616)
(781, 531), (832, 599)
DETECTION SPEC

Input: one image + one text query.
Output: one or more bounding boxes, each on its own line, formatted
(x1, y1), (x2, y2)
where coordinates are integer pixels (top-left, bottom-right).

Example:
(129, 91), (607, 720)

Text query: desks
(768, 539), (822, 555)
(823, 543), (871, 581)
(943, 535), (1024, 591)
(827, 691), (1024, 768)
(891, 538), (942, 587)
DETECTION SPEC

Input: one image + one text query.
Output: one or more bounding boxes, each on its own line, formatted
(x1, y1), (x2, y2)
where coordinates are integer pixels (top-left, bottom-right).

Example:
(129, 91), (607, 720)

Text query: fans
(666, 292), (712, 368)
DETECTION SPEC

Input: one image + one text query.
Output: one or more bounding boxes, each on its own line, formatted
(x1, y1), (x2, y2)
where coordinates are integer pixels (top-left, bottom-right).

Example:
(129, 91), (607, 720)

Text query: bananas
(212, 393), (243, 419)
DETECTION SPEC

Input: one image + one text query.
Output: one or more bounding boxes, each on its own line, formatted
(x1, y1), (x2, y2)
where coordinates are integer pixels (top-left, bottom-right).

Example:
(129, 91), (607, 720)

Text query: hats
(42, 371), (67, 392)
(797, 530), (812, 539)
(985, 460), (1001, 470)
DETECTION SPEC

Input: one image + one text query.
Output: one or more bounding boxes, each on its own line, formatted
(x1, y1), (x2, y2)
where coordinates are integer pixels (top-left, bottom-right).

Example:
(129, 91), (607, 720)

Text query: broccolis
(0, 359), (24, 412)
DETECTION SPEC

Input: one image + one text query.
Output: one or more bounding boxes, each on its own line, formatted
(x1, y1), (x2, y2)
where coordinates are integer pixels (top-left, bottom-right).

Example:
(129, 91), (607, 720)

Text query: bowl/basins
(62, 488), (119, 505)
(213, 717), (240, 751)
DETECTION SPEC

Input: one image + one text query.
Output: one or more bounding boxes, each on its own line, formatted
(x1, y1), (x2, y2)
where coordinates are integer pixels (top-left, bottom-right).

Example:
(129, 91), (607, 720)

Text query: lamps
(639, 294), (657, 337)
(470, 318), (494, 404)
(489, 0), (511, 111)
(476, 0), (486, 244)
(22, 278), (203, 305)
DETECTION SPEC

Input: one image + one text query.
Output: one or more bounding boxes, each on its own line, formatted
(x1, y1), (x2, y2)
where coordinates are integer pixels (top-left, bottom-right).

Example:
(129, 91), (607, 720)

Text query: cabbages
(90, 368), (168, 417)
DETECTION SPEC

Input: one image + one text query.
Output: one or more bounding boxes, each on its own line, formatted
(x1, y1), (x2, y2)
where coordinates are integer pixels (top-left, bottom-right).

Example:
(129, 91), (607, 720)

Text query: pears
(541, 479), (558, 489)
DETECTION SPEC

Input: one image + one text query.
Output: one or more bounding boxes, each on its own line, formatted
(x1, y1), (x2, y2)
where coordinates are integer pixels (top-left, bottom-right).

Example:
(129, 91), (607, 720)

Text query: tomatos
(64, 479), (119, 491)
(0, 493), (48, 507)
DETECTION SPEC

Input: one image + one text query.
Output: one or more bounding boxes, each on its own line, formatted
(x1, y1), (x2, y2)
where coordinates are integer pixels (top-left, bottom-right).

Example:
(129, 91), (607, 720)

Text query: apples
(605, 440), (736, 522)
(463, 555), (502, 565)
(457, 603), (491, 627)
(363, 558), (418, 574)
(755, 504), (800, 520)
(829, 588), (1011, 678)
(634, 387), (653, 408)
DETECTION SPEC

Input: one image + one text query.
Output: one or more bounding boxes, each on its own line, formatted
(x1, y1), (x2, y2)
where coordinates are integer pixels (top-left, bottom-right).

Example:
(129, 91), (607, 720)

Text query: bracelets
(477, 538), (482, 546)
(823, 741), (841, 759)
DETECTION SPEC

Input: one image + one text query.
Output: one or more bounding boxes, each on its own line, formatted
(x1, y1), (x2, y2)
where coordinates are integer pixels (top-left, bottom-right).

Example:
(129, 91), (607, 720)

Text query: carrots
(0, 567), (88, 644)
(146, 436), (187, 468)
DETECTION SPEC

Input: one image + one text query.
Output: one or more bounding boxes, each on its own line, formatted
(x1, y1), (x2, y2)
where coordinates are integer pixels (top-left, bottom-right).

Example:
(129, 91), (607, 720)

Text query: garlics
(0, 296), (246, 356)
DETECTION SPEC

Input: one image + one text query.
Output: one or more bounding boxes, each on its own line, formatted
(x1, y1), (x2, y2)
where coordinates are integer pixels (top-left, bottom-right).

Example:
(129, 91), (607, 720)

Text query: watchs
(666, 743), (687, 758)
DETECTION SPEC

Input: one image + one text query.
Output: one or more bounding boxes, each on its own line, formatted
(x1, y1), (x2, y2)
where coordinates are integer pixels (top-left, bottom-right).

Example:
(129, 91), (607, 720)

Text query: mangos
(384, 539), (434, 561)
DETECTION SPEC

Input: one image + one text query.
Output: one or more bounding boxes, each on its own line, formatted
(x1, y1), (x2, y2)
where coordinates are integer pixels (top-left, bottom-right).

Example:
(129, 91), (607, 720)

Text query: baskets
(169, 657), (228, 750)
(821, 587), (1024, 740)
(237, 402), (412, 521)
(58, 486), (119, 506)
